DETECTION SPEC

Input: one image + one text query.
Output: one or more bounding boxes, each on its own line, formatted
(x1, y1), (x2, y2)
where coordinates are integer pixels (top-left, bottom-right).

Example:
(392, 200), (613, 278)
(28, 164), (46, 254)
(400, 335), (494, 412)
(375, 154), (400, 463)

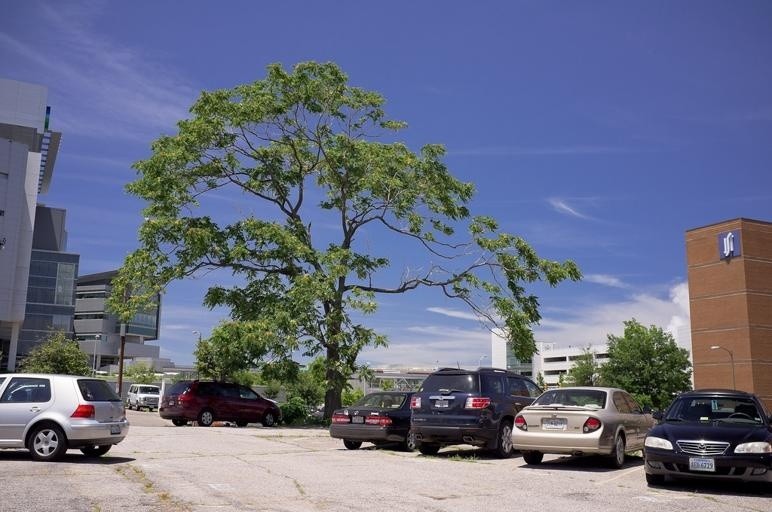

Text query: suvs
(410, 363), (547, 459)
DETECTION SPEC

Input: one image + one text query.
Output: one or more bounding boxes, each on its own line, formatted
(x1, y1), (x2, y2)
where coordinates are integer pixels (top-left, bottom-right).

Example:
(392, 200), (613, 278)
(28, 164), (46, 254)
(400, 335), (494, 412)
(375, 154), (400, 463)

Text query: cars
(329, 391), (420, 453)
(508, 384), (652, 466)
(645, 390), (772, 493)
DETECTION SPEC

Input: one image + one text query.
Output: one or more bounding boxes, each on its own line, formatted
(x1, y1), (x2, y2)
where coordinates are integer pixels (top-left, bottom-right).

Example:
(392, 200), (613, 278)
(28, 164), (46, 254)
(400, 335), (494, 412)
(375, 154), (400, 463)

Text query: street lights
(92, 335), (101, 377)
(191, 330), (203, 380)
(709, 345), (737, 393)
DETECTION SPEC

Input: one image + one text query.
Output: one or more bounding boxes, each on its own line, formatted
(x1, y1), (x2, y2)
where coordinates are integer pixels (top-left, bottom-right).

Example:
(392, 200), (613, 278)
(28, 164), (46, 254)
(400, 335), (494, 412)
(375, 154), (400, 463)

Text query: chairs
(688, 402), (757, 419)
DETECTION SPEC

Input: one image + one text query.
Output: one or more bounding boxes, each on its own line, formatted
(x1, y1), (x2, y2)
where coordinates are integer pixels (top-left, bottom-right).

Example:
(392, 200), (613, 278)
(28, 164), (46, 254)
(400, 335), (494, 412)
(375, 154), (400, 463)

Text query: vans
(157, 378), (285, 427)
(126, 384), (162, 412)
(0, 374), (129, 458)
